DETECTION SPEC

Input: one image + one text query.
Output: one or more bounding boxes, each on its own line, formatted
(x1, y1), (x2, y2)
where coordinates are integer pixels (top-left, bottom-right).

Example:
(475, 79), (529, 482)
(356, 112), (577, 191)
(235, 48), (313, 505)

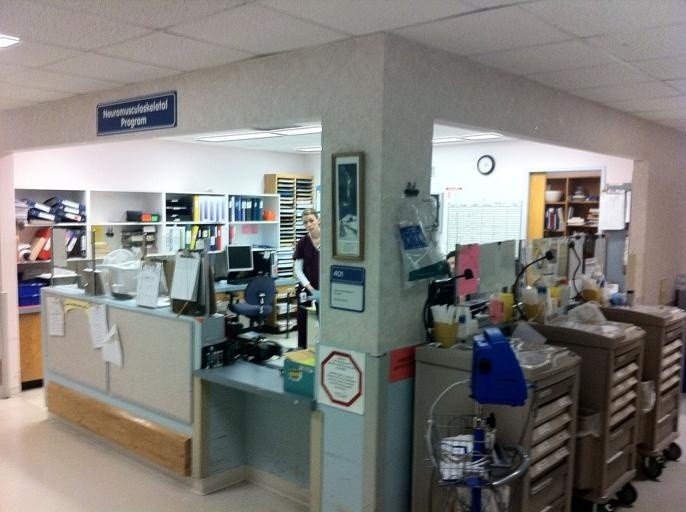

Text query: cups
(433, 321), (459, 348)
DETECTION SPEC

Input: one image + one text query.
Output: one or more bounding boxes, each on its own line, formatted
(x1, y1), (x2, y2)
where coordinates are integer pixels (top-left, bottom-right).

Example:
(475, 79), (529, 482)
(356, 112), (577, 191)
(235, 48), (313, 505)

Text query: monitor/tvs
(227, 245), (253, 276)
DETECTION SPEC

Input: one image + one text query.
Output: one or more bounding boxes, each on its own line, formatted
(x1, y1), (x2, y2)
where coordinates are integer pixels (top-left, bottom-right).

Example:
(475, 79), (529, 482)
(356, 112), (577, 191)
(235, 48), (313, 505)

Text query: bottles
(498, 286), (514, 325)
(626, 288), (634, 308)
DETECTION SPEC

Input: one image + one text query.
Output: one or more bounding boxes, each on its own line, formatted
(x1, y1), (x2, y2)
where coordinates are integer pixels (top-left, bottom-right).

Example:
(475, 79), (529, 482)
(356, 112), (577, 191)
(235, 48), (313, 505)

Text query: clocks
(476, 155), (494, 175)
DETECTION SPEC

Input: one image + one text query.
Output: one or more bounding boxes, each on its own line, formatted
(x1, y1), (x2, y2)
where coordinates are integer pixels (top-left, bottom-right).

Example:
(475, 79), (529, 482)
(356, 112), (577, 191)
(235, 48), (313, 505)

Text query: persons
(446, 251), (456, 278)
(292, 209), (321, 349)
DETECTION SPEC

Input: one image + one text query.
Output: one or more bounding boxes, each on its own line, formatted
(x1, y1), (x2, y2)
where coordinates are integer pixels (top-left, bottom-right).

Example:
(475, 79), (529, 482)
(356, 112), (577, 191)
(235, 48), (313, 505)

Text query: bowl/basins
(546, 190), (563, 202)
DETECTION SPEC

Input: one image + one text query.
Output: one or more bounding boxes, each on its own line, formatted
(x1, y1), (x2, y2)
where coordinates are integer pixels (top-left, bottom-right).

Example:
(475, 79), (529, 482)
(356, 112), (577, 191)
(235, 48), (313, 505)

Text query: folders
(34, 227), (86, 260)
(235, 197), (264, 221)
(20, 196), (86, 224)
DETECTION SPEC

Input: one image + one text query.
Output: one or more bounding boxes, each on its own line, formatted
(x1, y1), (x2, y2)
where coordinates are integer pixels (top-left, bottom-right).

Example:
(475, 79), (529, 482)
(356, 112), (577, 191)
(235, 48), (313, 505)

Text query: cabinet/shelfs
(14, 173), (317, 392)
(523, 168), (603, 287)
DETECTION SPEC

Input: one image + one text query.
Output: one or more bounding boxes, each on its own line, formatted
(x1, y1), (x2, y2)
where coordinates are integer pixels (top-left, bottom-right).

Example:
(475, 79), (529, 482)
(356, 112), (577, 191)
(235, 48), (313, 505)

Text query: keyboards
(228, 277), (254, 284)
